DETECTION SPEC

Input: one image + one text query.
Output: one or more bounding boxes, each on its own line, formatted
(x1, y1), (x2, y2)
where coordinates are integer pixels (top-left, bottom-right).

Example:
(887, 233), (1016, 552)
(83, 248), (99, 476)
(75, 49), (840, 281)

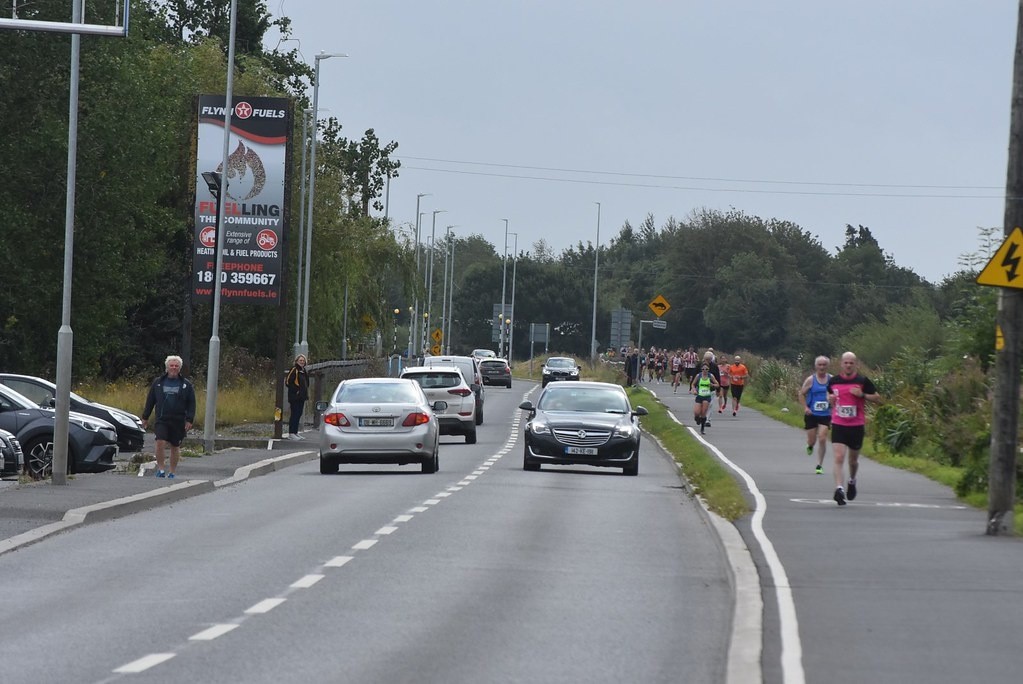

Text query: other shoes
(673, 390), (677, 394)
(671, 381), (674, 386)
(736, 403), (740, 411)
(705, 422), (711, 427)
(733, 411), (736, 416)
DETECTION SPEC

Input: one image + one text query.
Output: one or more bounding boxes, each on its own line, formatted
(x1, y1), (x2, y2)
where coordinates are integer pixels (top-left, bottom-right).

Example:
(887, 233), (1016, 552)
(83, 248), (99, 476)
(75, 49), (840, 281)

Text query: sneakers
(834, 488), (846, 505)
(156, 470), (166, 477)
(700, 429), (706, 434)
(806, 446), (813, 455)
(847, 481), (856, 500)
(167, 473), (175, 478)
(815, 465), (823, 474)
(697, 418), (700, 424)
(287, 435), (305, 440)
(718, 404), (725, 413)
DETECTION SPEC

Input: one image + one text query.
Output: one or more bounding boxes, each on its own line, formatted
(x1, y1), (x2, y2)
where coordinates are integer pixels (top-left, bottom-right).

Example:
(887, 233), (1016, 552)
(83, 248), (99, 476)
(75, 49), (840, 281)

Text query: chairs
(442, 376), (454, 386)
(412, 376), (423, 387)
(426, 380), (436, 386)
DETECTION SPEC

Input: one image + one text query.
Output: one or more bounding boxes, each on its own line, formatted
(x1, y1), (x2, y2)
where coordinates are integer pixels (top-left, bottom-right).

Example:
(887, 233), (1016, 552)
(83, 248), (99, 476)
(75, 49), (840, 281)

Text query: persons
(799, 356), (834, 475)
(141, 356), (196, 478)
(826, 351), (880, 506)
(606, 344), (749, 435)
(285, 354), (309, 441)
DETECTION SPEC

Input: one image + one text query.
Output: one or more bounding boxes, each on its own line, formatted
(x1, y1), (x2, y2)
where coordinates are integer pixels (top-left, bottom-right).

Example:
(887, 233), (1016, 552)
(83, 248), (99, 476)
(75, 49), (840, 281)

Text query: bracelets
(861, 392), (866, 399)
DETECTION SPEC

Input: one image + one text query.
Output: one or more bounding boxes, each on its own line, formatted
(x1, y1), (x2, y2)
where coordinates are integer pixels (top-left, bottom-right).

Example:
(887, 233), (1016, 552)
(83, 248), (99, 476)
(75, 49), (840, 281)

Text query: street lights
(591, 202), (601, 356)
(293, 49), (351, 363)
(407, 193), (518, 376)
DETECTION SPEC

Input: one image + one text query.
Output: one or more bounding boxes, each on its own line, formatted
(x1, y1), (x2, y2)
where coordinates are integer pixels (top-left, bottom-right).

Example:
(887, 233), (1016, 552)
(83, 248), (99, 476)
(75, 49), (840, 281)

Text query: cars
(0, 428), (25, 480)
(519, 380), (649, 477)
(0, 373), (147, 453)
(0, 382), (119, 481)
(315, 377), (441, 475)
(470, 349), (500, 364)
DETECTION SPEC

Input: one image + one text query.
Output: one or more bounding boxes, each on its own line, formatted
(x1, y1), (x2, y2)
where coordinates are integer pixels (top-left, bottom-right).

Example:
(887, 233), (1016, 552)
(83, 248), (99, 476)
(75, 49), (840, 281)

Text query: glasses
(701, 368), (709, 371)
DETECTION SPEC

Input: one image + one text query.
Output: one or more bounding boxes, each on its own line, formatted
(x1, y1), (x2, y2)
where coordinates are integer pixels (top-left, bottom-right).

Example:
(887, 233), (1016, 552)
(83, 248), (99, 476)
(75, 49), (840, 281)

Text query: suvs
(540, 356), (582, 389)
(398, 365), (476, 445)
(477, 358), (513, 389)
(421, 356), (485, 426)
